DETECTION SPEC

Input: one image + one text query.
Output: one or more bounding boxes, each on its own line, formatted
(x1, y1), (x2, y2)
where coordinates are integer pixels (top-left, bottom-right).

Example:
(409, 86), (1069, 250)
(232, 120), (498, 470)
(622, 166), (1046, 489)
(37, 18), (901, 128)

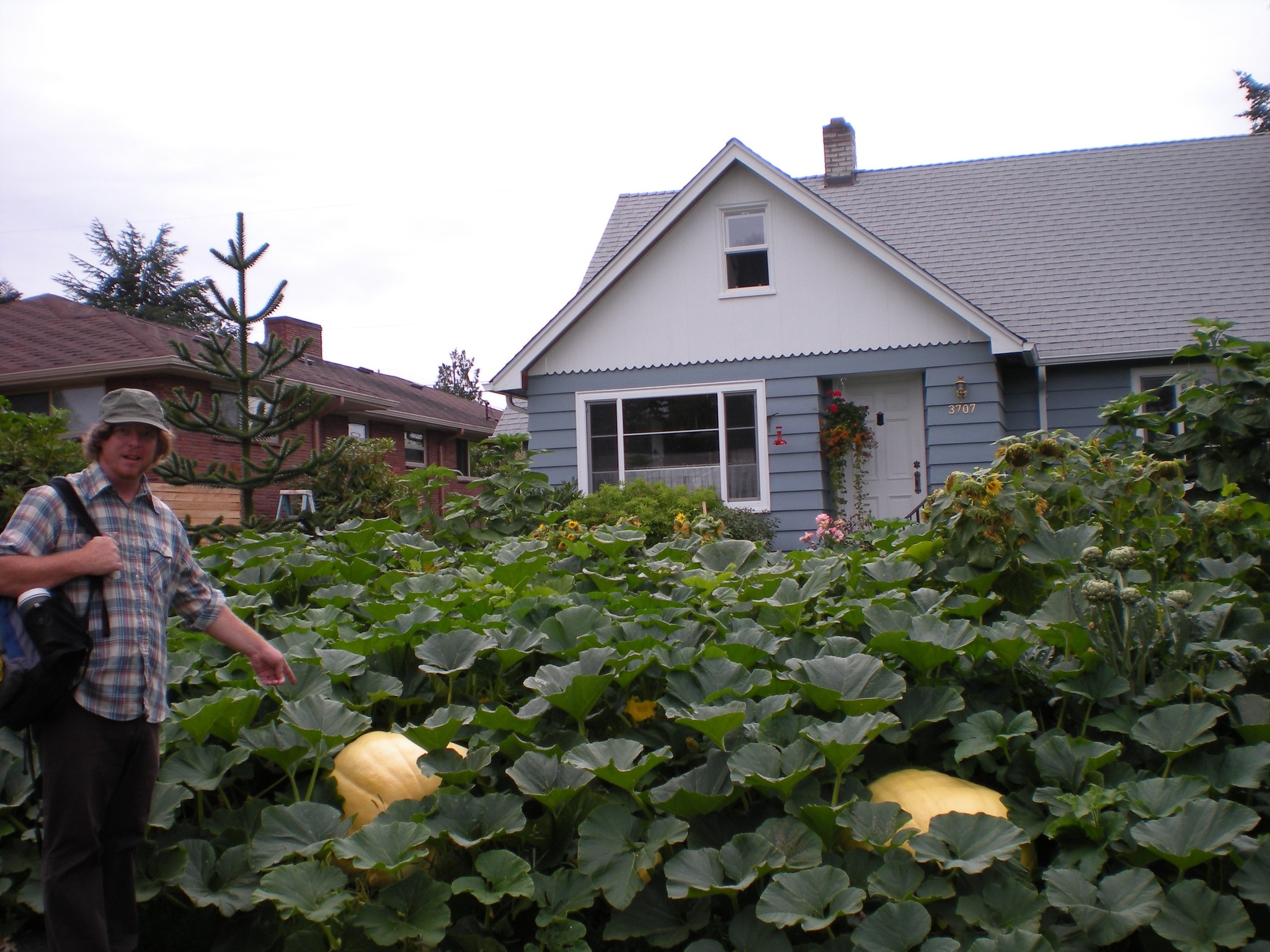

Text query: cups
(17, 587), (57, 645)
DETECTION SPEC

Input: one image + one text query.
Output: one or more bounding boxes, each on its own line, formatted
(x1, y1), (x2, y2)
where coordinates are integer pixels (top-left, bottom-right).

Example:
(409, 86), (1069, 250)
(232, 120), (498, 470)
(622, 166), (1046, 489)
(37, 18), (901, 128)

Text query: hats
(97, 387), (169, 433)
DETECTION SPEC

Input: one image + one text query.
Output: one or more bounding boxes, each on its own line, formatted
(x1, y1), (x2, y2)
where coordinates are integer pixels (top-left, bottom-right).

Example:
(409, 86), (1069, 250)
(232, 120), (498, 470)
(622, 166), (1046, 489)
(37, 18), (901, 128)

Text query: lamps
(766, 412), (787, 445)
(954, 375), (967, 404)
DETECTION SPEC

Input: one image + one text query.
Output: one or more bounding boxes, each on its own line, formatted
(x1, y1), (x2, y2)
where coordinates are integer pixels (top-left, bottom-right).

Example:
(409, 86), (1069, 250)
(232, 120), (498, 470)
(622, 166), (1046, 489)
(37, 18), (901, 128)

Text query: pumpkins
(842, 768), (1037, 874)
(326, 730), (468, 883)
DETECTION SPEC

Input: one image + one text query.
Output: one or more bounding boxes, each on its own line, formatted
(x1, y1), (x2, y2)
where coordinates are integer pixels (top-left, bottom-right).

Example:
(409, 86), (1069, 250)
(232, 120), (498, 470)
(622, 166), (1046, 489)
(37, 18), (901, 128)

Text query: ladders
(277, 488), (314, 519)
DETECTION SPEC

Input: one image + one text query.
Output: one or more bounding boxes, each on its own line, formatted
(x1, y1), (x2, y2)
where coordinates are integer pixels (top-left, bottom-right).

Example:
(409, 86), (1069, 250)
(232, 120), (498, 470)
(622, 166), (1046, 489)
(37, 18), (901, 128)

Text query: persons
(0, 388), (296, 952)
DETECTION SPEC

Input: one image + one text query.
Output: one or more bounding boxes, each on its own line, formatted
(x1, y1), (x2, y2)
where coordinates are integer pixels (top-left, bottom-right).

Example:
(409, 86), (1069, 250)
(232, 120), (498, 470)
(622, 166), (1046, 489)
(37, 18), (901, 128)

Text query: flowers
(816, 390), (878, 530)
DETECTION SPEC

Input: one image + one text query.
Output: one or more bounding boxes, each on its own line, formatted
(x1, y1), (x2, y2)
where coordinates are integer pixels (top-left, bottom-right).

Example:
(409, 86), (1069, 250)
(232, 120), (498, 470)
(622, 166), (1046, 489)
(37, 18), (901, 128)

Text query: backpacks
(0, 477), (110, 734)
